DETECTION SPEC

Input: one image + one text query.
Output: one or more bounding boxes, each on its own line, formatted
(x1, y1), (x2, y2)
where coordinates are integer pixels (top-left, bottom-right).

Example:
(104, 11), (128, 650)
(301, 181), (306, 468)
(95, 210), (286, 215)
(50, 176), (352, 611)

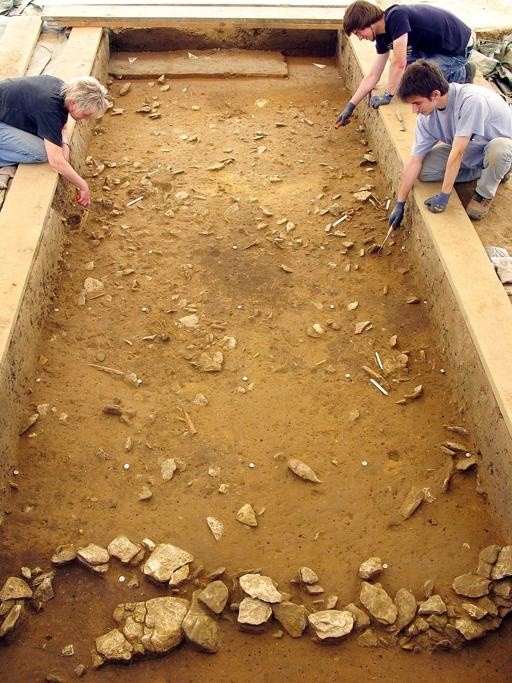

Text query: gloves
(424, 191), (450, 213)
(335, 101), (356, 126)
(369, 90), (392, 109)
(389, 202), (405, 230)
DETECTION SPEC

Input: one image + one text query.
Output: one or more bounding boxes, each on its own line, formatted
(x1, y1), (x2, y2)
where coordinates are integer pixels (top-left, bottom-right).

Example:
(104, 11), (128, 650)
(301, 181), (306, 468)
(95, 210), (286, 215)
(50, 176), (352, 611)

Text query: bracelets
(62, 141), (70, 147)
(384, 89), (394, 96)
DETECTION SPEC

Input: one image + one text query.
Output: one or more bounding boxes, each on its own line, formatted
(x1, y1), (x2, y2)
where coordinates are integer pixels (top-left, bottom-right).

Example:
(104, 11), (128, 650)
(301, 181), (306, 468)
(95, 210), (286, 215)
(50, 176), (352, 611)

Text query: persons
(387, 60), (512, 230)
(332, 0), (477, 127)
(1, 74), (110, 208)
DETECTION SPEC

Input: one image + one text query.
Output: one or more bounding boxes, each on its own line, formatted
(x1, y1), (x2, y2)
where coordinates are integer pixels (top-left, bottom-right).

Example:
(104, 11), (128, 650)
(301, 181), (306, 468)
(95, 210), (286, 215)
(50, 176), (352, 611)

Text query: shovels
(323, 121), (345, 138)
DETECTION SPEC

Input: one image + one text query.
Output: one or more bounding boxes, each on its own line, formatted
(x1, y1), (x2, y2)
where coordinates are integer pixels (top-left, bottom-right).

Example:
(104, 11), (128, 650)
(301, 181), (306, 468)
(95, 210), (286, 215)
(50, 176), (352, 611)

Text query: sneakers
(466, 190), (493, 220)
(465, 61), (477, 82)
